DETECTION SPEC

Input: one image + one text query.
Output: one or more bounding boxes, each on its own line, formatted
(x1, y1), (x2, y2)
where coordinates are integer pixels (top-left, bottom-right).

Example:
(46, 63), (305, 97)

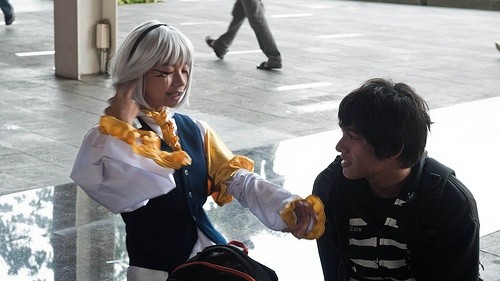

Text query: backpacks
(167, 241), (278, 281)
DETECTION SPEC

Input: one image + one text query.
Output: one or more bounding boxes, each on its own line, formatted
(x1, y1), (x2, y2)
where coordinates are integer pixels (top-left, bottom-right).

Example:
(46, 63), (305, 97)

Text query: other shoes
(6, 16), (14, 25)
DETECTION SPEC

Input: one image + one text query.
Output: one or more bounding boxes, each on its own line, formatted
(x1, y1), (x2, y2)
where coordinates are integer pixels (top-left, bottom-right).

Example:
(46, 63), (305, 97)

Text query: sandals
(206, 36), (224, 60)
(257, 61), (281, 69)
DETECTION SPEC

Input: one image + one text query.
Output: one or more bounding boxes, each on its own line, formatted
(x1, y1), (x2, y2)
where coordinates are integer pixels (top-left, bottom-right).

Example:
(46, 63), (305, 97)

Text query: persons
(0, 0), (15, 25)
(206, 0), (282, 70)
(312, 78), (485, 281)
(69, 21), (326, 281)
